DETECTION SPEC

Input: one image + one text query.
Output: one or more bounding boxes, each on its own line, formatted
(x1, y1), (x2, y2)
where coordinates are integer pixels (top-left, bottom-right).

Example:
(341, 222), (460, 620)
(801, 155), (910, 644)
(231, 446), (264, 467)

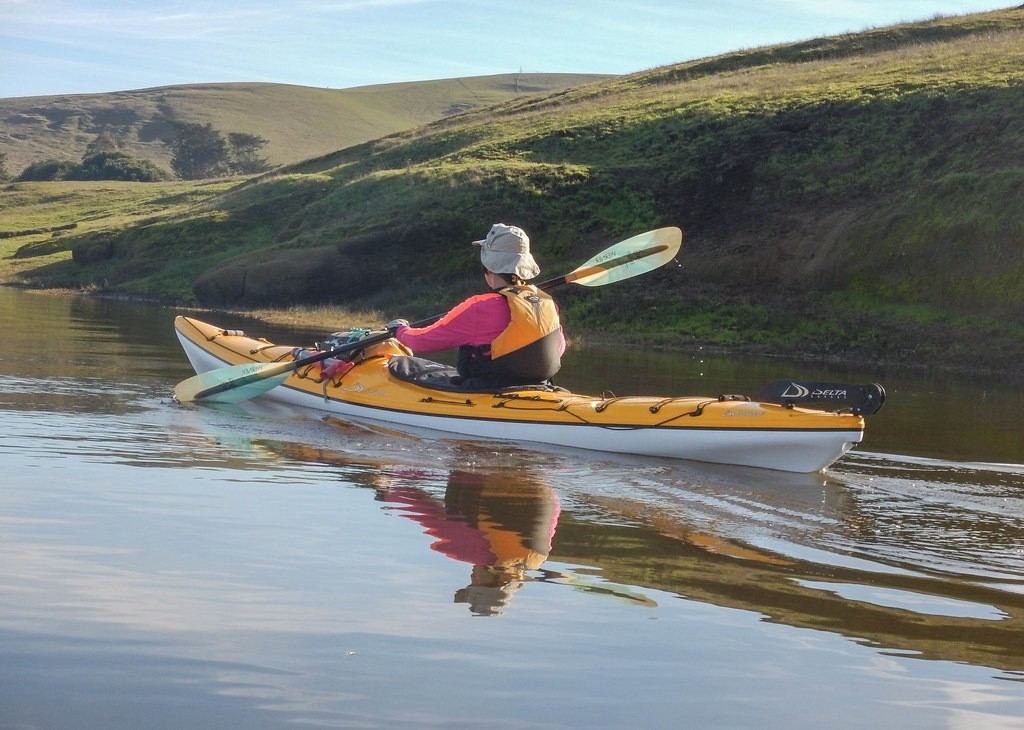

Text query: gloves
(385, 319), (410, 338)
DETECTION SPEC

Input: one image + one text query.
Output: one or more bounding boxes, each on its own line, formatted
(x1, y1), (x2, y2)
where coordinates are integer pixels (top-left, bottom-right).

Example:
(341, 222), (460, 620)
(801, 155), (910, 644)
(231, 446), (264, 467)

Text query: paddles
(171, 225), (685, 406)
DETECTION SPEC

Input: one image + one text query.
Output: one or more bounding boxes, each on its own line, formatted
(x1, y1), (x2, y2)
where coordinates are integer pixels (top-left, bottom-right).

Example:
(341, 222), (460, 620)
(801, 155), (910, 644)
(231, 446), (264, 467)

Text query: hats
(471, 223), (541, 281)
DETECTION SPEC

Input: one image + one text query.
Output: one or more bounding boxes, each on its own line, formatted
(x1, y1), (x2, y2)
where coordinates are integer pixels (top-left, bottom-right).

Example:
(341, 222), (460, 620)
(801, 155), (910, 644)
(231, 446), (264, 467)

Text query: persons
(376, 439), (563, 617)
(383, 222), (567, 391)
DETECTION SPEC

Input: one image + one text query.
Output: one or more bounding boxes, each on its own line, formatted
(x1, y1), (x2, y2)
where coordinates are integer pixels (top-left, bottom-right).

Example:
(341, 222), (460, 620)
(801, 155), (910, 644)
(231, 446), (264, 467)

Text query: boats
(173, 316), (886, 474)
